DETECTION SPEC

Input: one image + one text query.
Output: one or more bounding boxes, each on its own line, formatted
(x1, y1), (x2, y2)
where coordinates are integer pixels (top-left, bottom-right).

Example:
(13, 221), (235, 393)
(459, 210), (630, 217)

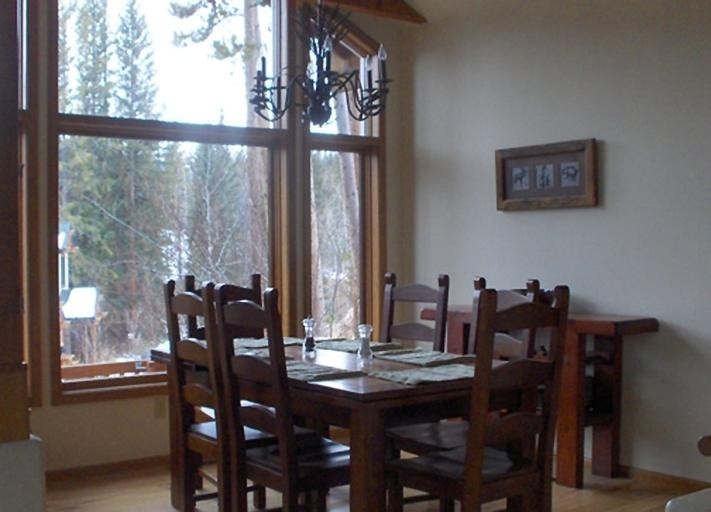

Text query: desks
(418, 304), (658, 488)
(149, 333), (512, 510)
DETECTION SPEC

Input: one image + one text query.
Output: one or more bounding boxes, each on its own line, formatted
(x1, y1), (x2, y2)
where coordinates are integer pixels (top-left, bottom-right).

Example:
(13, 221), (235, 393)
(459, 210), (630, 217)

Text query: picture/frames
(495, 136), (598, 211)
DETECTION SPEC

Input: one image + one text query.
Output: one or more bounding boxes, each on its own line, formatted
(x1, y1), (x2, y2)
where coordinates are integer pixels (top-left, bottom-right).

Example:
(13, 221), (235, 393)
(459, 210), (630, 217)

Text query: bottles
(356, 323), (374, 360)
(301, 318), (317, 355)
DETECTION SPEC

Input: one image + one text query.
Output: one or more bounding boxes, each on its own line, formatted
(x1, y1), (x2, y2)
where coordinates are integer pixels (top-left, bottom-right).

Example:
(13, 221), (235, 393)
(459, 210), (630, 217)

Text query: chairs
(665, 435), (711, 512)
(381, 268), (571, 512)
(158, 273), (353, 512)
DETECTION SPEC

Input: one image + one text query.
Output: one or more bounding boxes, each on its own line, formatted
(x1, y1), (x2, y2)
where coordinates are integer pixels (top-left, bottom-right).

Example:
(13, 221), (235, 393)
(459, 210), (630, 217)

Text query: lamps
(250, 0), (393, 123)
